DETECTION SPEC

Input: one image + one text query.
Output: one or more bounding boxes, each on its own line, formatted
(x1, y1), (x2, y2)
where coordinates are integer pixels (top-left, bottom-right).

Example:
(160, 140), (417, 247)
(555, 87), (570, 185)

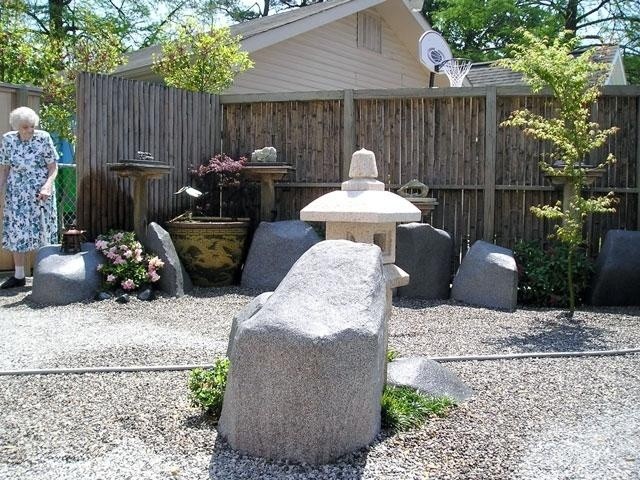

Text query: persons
(0, 106), (60, 289)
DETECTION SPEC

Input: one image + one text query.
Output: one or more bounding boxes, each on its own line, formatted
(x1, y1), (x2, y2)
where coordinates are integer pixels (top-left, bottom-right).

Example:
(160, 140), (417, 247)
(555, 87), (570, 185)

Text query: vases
(164, 221), (249, 287)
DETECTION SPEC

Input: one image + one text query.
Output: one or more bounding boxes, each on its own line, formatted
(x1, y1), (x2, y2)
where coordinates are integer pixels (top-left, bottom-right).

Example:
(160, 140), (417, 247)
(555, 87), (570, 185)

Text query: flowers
(188, 153), (248, 217)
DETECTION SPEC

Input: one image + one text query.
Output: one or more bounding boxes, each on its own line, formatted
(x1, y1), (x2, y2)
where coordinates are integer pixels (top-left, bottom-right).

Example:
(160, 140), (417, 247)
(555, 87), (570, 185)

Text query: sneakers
(1, 275), (27, 290)
